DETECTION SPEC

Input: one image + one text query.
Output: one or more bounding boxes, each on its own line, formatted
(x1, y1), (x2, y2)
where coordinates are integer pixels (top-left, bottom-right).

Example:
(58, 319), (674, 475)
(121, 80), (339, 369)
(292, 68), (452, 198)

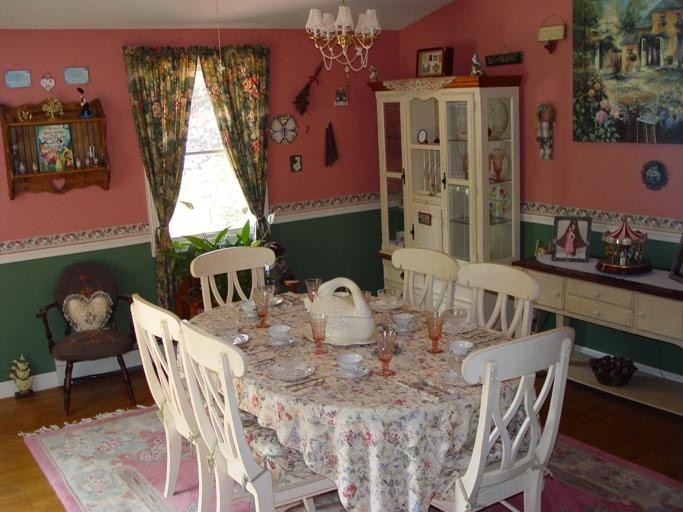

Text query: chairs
(391, 247), (459, 315)
(190, 246), (276, 311)
(36, 261), (137, 416)
(427, 326), (576, 512)
(129, 293), (210, 512)
(181, 318), (338, 512)
(458, 262), (541, 340)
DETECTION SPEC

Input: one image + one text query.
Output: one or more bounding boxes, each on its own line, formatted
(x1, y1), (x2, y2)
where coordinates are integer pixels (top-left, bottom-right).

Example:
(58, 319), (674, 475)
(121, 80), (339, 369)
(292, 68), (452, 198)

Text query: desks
(510, 255), (683, 417)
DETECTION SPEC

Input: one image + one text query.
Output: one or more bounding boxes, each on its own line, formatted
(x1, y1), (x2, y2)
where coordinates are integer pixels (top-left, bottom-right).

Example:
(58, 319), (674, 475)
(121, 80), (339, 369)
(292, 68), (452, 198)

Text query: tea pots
(305, 277), (376, 343)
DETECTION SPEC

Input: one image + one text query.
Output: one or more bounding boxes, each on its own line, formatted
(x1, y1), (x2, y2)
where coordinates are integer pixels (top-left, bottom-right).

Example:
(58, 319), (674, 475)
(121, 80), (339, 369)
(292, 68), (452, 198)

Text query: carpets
(18, 403), (683, 512)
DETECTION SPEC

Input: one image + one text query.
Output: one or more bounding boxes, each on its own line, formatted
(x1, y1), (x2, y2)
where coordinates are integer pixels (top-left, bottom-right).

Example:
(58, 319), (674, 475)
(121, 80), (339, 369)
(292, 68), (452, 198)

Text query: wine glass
(309, 313), (329, 355)
(426, 311), (445, 354)
(306, 279), (323, 301)
(253, 287), (271, 328)
(493, 151), (504, 182)
(377, 329), (396, 377)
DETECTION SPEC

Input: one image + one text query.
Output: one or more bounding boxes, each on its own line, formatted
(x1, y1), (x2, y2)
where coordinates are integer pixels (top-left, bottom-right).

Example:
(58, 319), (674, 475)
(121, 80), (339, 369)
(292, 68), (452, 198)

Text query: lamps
(305, 0), (381, 72)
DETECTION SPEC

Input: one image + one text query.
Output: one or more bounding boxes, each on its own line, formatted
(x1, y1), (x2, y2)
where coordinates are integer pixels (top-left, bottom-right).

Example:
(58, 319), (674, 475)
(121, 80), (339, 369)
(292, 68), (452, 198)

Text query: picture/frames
(570, 0), (683, 145)
(553, 215), (593, 263)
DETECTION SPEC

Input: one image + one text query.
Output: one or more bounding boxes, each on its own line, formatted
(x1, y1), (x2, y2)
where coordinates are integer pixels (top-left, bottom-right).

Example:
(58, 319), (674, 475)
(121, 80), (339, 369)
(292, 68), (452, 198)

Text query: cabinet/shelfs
(3, 97), (110, 199)
(366, 73), (525, 331)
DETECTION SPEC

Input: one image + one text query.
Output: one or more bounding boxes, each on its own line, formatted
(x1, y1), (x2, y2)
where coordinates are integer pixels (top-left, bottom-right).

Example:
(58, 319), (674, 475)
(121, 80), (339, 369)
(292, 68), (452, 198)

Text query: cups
(269, 326), (292, 341)
(212, 320), (241, 342)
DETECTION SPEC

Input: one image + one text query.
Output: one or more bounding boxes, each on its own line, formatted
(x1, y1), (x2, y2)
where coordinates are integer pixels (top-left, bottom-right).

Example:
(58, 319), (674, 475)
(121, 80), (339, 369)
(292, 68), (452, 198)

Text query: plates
(301, 327), (382, 347)
(390, 324), (421, 336)
(488, 99), (509, 139)
(441, 367), (476, 388)
(242, 297), (284, 309)
(265, 338), (297, 346)
(219, 334), (248, 345)
(333, 369), (370, 379)
(268, 364), (315, 383)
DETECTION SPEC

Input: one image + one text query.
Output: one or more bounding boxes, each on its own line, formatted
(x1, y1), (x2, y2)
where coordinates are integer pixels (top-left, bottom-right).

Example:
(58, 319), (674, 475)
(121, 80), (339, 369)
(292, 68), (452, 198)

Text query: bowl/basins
(449, 339), (475, 355)
(590, 356), (639, 387)
(337, 354), (364, 371)
(392, 314), (414, 329)
(377, 288), (403, 307)
